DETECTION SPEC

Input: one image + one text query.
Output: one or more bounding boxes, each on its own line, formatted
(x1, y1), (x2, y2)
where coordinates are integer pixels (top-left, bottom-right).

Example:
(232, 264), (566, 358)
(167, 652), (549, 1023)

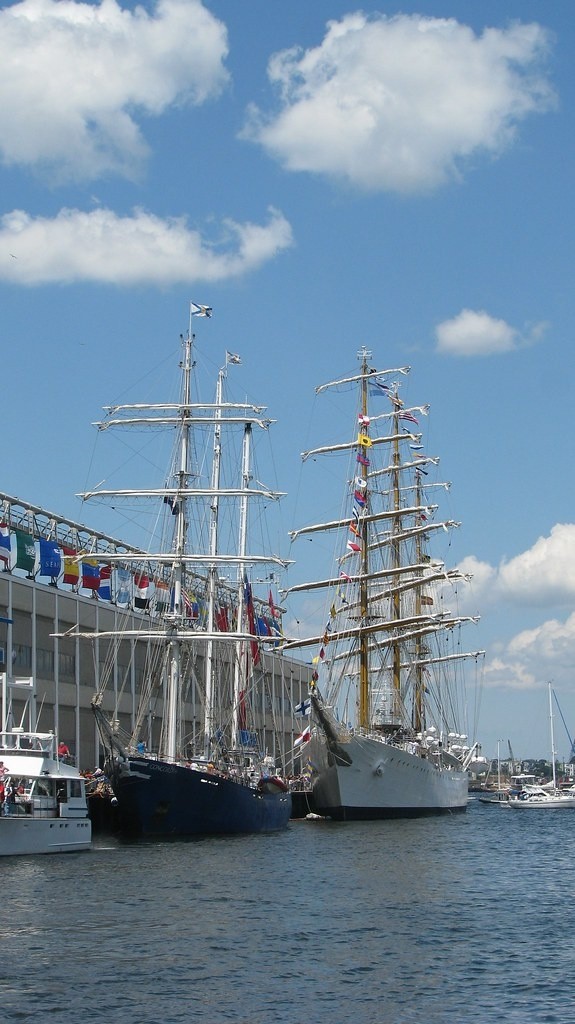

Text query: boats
(467, 793), (477, 800)
(80, 760), (126, 837)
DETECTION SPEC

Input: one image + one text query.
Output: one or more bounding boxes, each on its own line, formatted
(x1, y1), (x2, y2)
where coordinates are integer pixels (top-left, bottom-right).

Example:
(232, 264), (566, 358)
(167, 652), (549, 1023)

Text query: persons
(0, 761), (20, 814)
(136, 740), (145, 755)
(93, 766), (104, 778)
(55, 742), (70, 763)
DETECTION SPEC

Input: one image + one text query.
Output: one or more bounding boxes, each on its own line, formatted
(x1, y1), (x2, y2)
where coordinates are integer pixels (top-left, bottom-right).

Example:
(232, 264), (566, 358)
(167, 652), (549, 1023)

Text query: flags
(388, 369), (434, 696)
(0, 520), (284, 681)
(293, 726), (311, 749)
(302, 756), (315, 781)
(227, 350), (242, 365)
(191, 301), (212, 319)
(293, 698), (312, 716)
(368, 375), (392, 397)
(305, 412), (373, 684)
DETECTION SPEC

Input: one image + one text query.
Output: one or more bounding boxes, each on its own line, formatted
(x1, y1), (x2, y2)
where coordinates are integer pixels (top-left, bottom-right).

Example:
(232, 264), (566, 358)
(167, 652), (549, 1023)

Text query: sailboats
(286, 662), (323, 822)
(297, 342), (487, 819)
(47, 298), (290, 841)
(0, 578), (93, 856)
(478, 740), (541, 806)
(512, 677), (574, 810)
